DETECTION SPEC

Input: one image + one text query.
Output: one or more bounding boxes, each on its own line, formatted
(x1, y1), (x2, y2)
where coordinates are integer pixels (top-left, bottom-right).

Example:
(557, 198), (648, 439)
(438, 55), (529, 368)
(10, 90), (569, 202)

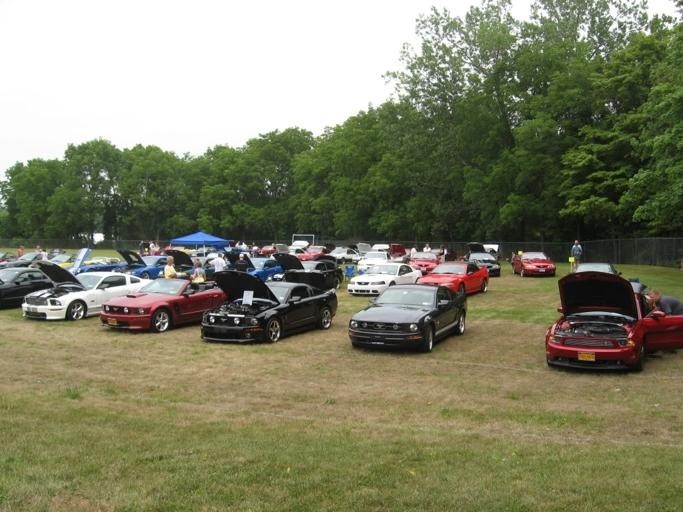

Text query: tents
(167, 232), (230, 257)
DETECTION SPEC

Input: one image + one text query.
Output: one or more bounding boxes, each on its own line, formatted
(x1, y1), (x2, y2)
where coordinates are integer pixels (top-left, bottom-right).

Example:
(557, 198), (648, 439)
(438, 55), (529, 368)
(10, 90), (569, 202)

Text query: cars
(158, 249), (216, 281)
(462, 241), (501, 261)
(0, 267), (54, 308)
(511, 251), (558, 277)
(575, 262), (623, 277)
(100, 277), (228, 334)
(256, 245), (276, 258)
(329, 247), (361, 265)
(64, 257), (130, 276)
(233, 253), (285, 282)
(21, 261), (154, 321)
(116, 247), (168, 280)
(272, 253), (343, 289)
(13, 252), (55, 267)
(49, 254), (94, 269)
(429, 248), (458, 259)
(288, 240), (310, 253)
(356, 252), (392, 275)
(408, 252), (442, 276)
(348, 284), (466, 353)
(545, 271), (683, 372)
(468, 252), (501, 277)
(200, 270), (337, 344)
(0, 251), (18, 269)
(415, 260), (490, 296)
(347, 262), (423, 297)
(297, 246), (332, 261)
(356, 242), (372, 257)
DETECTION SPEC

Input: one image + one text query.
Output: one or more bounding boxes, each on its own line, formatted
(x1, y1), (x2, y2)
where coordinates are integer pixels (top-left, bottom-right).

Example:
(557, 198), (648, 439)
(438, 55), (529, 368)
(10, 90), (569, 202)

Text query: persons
(136, 239), (161, 256)
(411, 244), (418, 257)
(424, 243), (432, 253)
(439, 245), (446, 262)
(209, 252), (228, 271)
(17, 246), (25, 257)
(190, 262), (207, 282)
(649, 289), (683, 317)
(235, 253), (248, 271)
(571, 240), (583, 268)
(34, 244), (48, 262)
(234, 240), (255, 252)
(164, 255), (177, 277)
